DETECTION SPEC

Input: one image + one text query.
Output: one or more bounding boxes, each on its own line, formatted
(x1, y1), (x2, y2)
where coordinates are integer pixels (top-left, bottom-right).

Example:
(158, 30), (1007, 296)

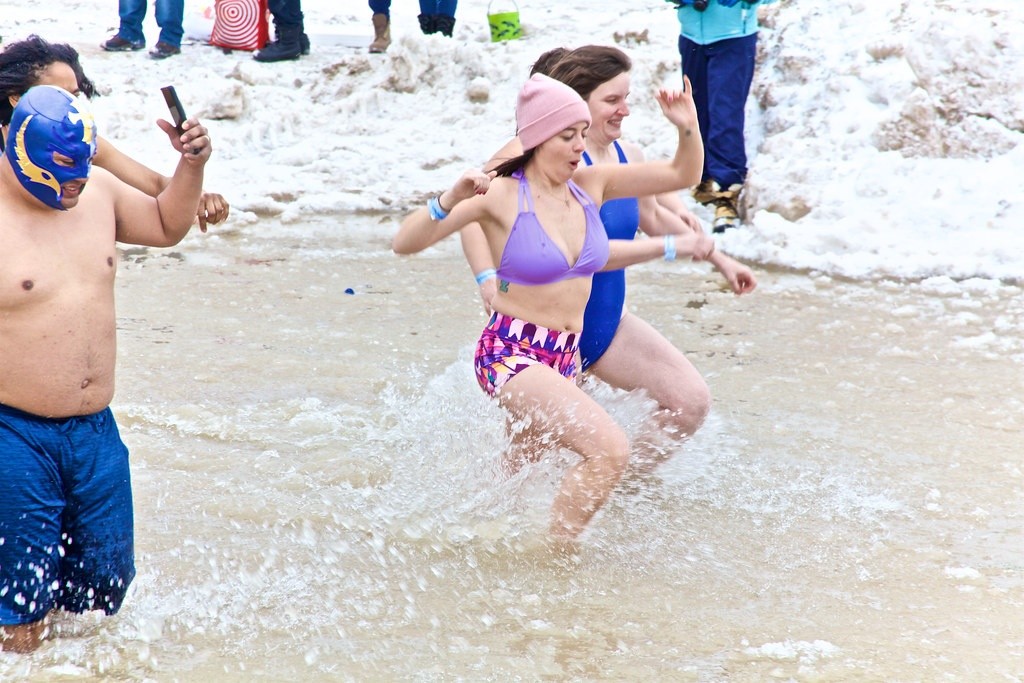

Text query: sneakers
(695, 176), (748, 233)
(149, 39), (181, 57)
(100, 34), (145, 52)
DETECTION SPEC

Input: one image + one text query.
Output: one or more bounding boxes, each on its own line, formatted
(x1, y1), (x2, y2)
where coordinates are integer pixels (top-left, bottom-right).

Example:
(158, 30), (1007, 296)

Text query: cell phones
(160, 86), (201, 155)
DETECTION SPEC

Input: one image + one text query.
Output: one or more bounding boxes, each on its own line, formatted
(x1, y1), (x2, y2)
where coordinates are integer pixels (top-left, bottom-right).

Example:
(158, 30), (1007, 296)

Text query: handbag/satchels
(208, 0), (269, 51)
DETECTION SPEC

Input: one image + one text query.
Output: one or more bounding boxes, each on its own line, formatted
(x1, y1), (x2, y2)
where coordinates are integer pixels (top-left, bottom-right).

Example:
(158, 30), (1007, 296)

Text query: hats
(513, 72), (592, 154)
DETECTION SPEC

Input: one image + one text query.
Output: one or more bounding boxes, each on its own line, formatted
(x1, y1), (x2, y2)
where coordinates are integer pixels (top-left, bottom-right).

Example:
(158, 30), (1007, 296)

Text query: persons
(666, 0), (765, 233)
(0, 33), (229, 655)
(460, 45), (758, 475)
(418, 0), (457, 38)
(252, 0), (310, 61)
(391, 72), (704, 548)
(100, 0), (184, 58)
(368, 0), (392, 52)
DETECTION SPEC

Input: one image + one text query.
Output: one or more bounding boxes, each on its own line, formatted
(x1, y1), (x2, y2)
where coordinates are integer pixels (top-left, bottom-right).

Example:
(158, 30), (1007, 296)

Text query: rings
(205, 135), (209, 141)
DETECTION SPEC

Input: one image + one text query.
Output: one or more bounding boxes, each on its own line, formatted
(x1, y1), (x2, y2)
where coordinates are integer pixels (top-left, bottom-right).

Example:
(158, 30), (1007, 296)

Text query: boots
(265, 13), (310, 55)
(251, 12), (302, 63)
(368, 11), (392, 53)
(417, 12), (455, 37)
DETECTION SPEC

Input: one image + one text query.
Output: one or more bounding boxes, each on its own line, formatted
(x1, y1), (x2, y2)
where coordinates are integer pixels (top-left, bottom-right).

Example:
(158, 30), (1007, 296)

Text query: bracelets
(428, 196), (451, 220)
(663, 235), (676, 261)
(475, 269), (496, 285)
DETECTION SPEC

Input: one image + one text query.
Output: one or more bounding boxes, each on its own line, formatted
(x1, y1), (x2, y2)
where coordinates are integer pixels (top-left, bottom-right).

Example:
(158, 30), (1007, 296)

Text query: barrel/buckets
(486, 0), (521, 42)
